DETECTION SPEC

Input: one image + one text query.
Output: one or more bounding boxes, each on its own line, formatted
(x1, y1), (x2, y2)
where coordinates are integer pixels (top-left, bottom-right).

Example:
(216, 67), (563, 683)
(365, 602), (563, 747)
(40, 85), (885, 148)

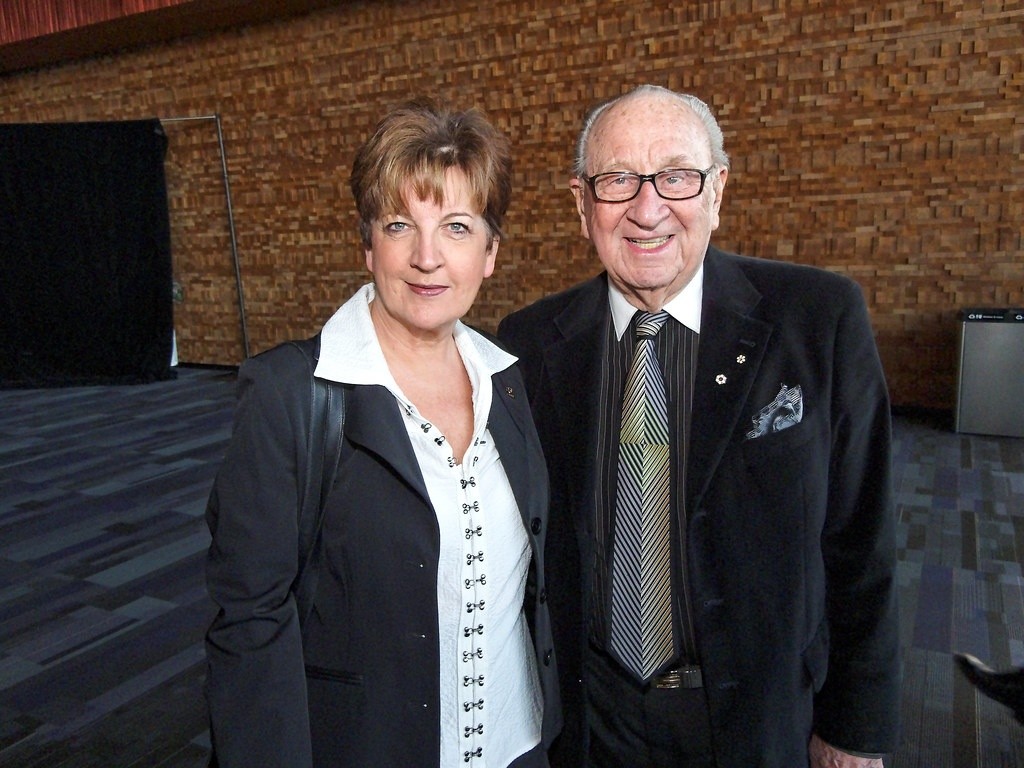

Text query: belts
(646, 667), (703, 690)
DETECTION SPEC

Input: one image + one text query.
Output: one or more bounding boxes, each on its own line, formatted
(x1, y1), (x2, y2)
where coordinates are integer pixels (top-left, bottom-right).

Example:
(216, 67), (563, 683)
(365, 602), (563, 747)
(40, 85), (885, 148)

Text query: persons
(496, 82), (907, 768)
(205, 95), (563, 767)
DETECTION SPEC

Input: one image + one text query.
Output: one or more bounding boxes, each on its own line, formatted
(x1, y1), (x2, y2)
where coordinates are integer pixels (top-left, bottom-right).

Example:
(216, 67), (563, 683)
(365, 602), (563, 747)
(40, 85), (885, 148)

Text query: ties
(604, 310), (681, 686)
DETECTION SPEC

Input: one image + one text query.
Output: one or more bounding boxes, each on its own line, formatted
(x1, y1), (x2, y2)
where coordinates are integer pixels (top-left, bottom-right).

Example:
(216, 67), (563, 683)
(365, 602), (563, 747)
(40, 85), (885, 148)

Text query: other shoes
(954, 649), (1024, 722)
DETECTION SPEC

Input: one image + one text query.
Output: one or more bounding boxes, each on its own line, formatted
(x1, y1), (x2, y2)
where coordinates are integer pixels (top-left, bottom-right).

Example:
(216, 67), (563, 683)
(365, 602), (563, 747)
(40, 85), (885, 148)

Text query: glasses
(582, 165), (714, 202)
